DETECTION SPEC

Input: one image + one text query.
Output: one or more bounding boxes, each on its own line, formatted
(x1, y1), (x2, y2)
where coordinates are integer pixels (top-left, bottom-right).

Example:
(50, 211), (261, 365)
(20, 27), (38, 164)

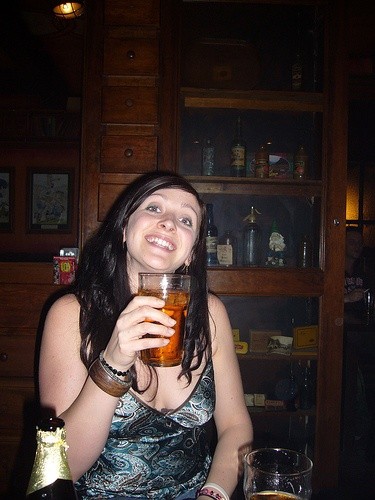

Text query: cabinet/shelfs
(0, 1), (346, 500)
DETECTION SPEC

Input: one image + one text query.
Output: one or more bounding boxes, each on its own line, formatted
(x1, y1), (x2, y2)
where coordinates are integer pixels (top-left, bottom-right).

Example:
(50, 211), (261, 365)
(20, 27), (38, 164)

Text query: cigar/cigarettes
(363, 289), (369, 293)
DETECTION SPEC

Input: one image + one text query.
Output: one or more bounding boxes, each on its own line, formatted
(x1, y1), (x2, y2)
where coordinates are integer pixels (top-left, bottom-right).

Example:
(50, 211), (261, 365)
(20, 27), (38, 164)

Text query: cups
(244, 449), (313, 500)
(363, 293), (374, 318)
(138, 273), (190, 367)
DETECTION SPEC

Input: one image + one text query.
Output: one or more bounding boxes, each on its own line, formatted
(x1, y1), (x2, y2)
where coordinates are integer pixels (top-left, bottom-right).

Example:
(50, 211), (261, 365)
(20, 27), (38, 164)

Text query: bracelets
(89, 350), (133, 397)
(197, 482), (231, 500)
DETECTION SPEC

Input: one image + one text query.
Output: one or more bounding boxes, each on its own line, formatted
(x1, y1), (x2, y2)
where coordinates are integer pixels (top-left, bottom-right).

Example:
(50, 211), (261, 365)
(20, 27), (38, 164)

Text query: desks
(344, 301), (375, 463)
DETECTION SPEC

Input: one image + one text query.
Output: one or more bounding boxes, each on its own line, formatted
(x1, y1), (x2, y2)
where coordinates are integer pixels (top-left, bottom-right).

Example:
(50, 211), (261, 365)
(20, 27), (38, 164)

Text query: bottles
(190, 141), (201, 176)
(221, 230), (234, 265)
(244, 213), (260, 266)
(299, 230), (312, 269)
(27, 417), (73, 500)
(203, 138), (215, 176)
(291, 46), (305, 90)
(256, 145), (268, 178)
(206, 204), (217, 265)
(231, 119), (247, 177)
(296, 117), (321, 180)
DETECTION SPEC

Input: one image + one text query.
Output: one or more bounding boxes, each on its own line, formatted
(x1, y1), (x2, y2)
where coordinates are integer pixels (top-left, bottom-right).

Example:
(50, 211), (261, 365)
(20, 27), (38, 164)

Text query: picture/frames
(27, 166), (75, 234)
(0, 165), (16, 233)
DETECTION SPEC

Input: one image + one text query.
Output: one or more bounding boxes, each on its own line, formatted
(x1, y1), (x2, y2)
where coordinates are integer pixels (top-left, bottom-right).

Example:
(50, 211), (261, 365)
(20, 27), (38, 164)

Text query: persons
(38, 172), (254, 500)
(344, 226), (375, 429)
